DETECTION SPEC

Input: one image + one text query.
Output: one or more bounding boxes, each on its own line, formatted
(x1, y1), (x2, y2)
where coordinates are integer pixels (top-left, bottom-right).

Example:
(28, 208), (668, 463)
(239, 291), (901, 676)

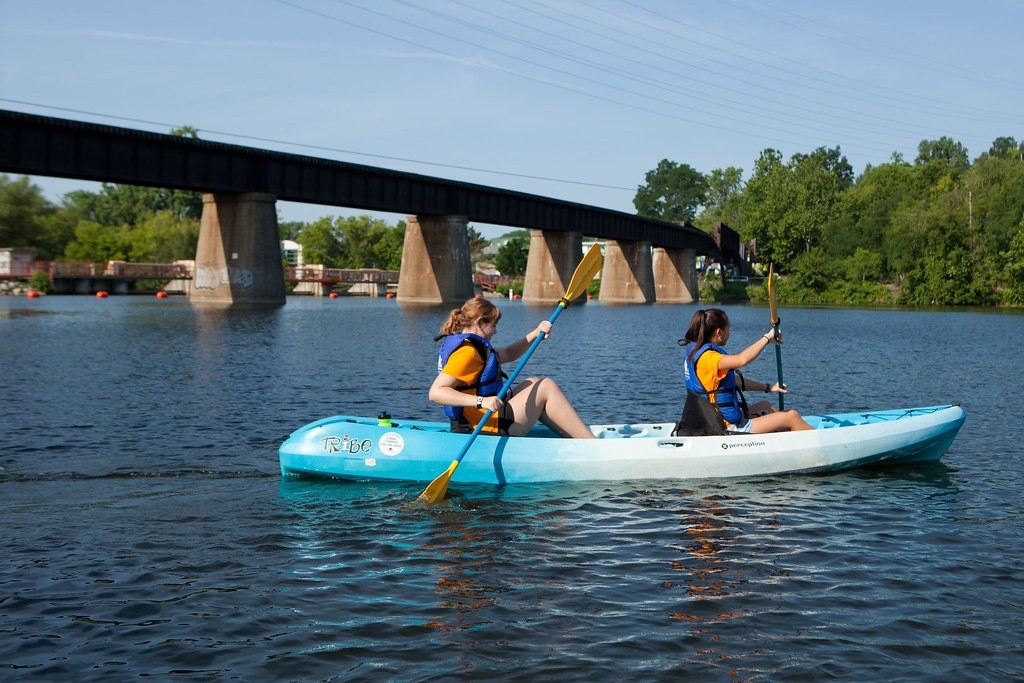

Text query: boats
(278, 403), (969, 485)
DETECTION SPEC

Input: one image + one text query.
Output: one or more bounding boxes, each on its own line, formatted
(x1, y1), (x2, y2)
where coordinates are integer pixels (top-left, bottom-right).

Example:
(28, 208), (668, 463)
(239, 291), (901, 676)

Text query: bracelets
(477, 396), (483, 408)
(765, 383), (771, 393)
(762, 334), (771, 343)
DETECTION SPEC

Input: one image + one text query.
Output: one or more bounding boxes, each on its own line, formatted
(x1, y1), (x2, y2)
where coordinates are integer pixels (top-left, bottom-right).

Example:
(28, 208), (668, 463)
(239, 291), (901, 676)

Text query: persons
(676, 308), (815, 437)
(428, 298), (597, 439)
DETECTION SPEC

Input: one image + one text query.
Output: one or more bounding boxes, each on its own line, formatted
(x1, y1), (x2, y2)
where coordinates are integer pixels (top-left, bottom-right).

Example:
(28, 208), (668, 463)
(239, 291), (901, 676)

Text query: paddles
(765, 260), (788, 411)
(418, 242), (604, 505)
